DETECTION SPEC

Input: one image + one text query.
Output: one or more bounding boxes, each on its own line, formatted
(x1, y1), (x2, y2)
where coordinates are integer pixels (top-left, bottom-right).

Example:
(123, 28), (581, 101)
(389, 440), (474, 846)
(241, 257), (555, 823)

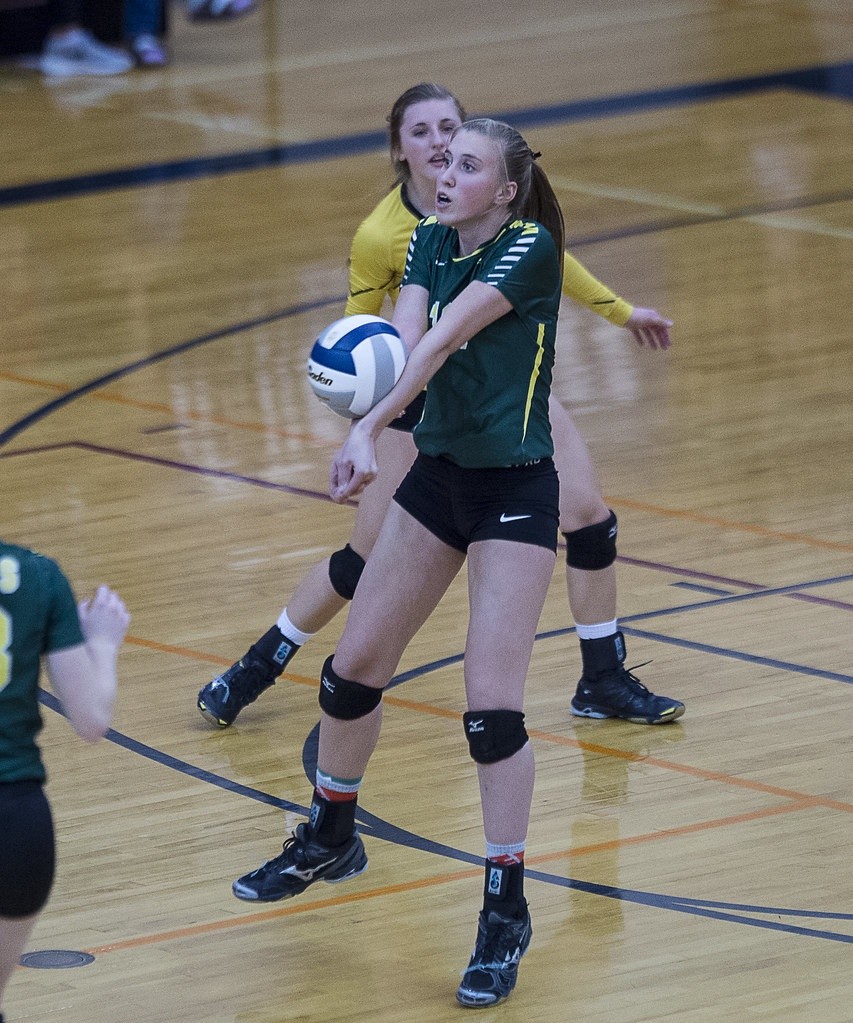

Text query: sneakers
(229, 822), (371, 903)
(572, 718), (685, 771)
(455, 898), (533, 1008)
(196, 646), (278, 730)
(569, 659), (685, 725)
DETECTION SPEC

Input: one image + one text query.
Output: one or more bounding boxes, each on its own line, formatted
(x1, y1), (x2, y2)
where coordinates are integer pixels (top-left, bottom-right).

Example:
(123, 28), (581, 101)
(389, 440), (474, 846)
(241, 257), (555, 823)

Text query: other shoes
(38, 28), (134, 77)
(130, 36), (170, 70)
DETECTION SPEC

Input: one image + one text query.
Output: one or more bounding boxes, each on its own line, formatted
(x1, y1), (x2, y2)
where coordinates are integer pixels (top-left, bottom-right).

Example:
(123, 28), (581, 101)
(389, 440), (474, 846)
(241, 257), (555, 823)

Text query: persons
(0, 541), (132, 1023)
(0, 0), (257, 75)
(235, 122), (566, 1006)
(198, 82), (687, 725)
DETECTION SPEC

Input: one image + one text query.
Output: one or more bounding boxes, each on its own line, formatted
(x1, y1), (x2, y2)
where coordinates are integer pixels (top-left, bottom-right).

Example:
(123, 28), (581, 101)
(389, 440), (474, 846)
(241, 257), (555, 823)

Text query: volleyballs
(305, 312), (409, 420)
